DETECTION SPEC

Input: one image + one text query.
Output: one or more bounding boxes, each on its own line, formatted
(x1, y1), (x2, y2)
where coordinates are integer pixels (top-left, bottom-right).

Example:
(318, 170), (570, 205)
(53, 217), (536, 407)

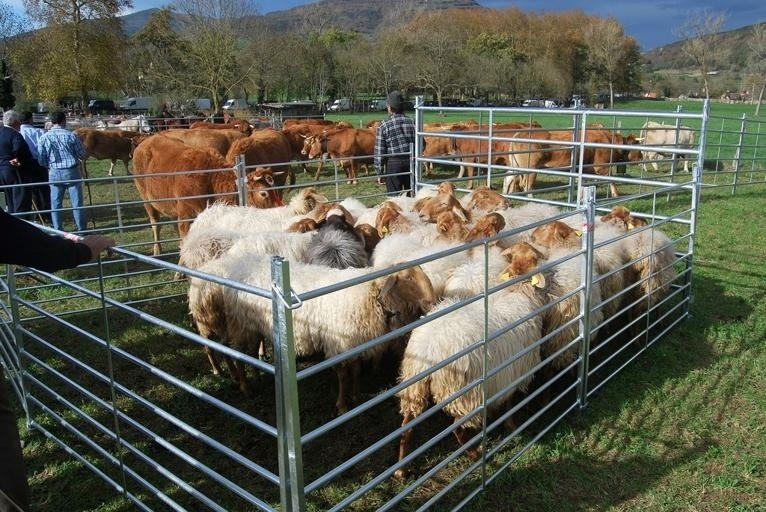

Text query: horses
(88, 108), (273, 132)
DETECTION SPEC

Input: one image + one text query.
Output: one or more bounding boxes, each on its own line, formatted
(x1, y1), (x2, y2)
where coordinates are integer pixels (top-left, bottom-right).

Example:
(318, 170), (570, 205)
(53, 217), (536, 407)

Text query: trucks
(522, 99), (540, 107)
(180, 99), (210, 110)
(221, 99), (247, 110)
(119, 96), (154, 109)
(539, 100), (558, 108)
(330, 98), (352, 111)
(367, 100), (386, 111)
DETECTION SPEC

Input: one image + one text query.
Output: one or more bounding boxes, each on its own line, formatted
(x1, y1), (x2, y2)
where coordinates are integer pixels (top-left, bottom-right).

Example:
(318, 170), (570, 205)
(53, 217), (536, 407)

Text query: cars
(570, 94), (603, 103)
(426, 96), (499, 108)
(37, 102), (50, 113)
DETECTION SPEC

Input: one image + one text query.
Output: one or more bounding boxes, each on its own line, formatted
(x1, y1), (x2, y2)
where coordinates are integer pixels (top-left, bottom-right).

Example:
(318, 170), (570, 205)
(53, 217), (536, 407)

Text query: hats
(386, 91), (405, 110)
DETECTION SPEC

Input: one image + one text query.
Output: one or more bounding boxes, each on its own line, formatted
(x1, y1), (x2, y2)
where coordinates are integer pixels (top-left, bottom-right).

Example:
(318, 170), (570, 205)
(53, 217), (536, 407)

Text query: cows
(154, 118), (385, 187)
(421, 120), (542, 189)
(638, 121), (697, 176)
(500, 123), (646, 204)
(132, 134), (286, 282)
(71, 127), (156, 177)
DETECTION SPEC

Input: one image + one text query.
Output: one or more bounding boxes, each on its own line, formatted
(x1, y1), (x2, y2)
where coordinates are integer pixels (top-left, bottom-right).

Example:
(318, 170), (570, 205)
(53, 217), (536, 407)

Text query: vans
(87, 99), (116, 109)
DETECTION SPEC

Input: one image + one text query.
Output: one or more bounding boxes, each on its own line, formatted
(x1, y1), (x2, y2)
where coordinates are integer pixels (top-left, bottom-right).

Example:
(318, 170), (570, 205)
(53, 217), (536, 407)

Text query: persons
(0, 206), (116, 512)
(374, 90), (426, 197)
(37, 111), (94, 235)
(18, 111), (52, 223)
(0, 110), (34, 221)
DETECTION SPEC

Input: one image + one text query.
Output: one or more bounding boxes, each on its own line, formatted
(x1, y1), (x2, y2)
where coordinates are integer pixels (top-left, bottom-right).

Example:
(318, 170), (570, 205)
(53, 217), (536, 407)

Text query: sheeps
(178, 181), (678, 484)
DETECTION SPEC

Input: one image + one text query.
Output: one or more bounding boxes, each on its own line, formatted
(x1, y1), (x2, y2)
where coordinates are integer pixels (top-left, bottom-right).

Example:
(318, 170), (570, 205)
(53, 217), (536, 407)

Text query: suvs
(59, 95), (80, 106)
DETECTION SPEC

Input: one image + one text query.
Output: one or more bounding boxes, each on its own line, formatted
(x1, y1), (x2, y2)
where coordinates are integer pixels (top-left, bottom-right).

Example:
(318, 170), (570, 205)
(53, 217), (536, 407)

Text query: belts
(388, 156), (410, 160)
